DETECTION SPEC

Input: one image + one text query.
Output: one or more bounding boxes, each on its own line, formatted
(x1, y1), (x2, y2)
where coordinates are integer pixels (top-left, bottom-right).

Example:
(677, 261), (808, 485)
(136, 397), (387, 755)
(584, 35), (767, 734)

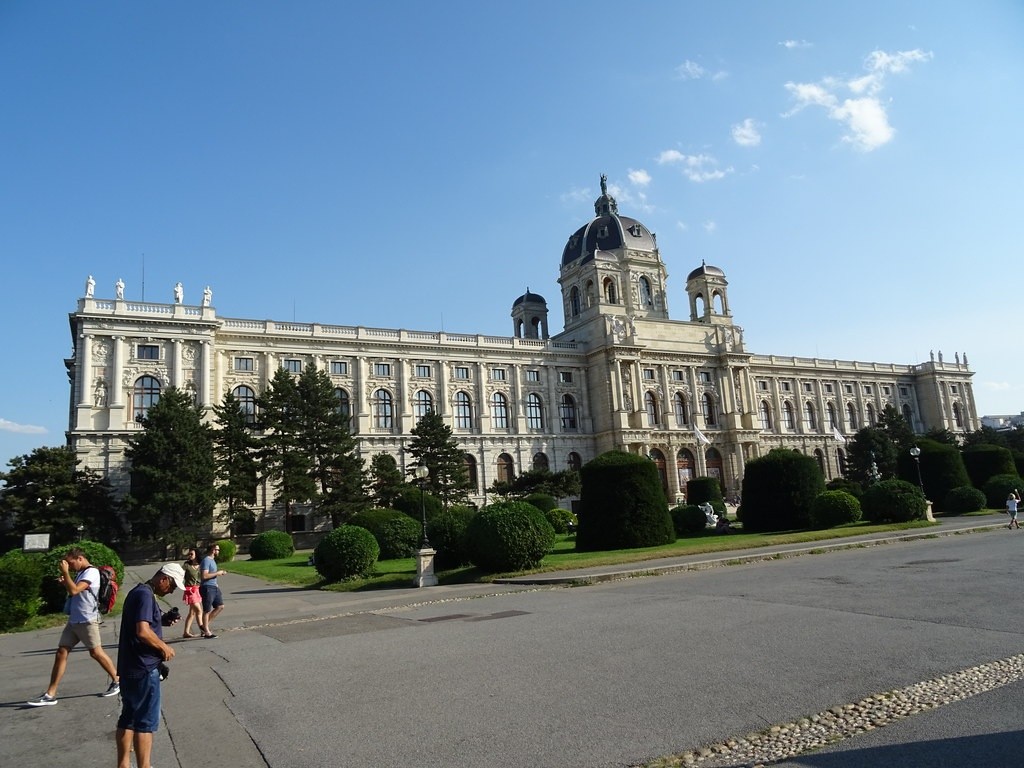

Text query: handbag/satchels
(64, 596), (71, 613)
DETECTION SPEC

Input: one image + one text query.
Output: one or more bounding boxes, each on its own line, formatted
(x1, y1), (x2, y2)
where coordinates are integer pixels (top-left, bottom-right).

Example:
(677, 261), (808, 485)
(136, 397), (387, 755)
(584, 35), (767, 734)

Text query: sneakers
(200, 625), (205, 633)
(101, 682), (120, 697)
(205, 634), (218, 639)
(27, 694), (58, 706)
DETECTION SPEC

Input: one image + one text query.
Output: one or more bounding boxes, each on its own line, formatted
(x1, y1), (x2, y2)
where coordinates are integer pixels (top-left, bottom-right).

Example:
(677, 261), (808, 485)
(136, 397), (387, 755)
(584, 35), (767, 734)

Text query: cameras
(161, 606), (180, 626)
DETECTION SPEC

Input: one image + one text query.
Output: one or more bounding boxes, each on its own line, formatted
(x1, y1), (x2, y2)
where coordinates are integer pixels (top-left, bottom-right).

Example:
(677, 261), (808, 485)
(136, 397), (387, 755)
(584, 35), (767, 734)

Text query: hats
(159, 563), (186, 591)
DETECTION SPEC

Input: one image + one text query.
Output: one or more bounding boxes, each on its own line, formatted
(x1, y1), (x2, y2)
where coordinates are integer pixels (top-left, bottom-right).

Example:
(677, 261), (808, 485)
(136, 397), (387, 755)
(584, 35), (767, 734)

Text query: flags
(694, 425), (711, 444)
(834, 427), (847, 441)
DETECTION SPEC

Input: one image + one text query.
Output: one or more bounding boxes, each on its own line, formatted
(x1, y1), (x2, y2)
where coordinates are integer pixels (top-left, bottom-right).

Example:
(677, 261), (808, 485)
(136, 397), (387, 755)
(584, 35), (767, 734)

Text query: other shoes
(201, 632), (205, 637)
(183, 633), (195, 637)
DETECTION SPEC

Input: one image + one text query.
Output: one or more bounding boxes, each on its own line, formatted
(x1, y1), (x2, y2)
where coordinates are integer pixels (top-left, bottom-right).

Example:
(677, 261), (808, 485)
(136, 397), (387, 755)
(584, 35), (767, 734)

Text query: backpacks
(81, 565), (118, 614)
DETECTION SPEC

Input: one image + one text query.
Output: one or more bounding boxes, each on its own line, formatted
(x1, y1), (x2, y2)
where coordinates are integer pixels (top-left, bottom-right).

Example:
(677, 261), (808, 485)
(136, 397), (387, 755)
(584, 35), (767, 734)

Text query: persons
(175, 283), (184, 303)
(1006, 494), (1023, 529)
(204, 286), (212, 302)
(115, 278), (125, 298)
(117, 563), (187, 768)
(182, 548), (212, 638)
(85, 275), (95, 296)
(27, 547), (120, 706)
(95, 383), (108, 405)
(199, 544), (227, 639)
(187, 386), (197, 407)
(730, 492), (741, 507)
(698, 502), (716, 526)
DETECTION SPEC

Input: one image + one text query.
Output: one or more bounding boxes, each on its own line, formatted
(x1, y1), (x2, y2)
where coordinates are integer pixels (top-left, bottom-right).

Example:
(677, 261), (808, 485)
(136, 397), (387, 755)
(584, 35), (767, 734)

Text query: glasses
(216, 549), (220, 552)
(189, 553), (194, 555)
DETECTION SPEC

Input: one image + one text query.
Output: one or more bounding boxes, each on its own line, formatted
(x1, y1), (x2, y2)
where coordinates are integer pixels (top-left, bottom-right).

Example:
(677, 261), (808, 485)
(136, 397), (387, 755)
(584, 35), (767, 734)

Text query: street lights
(414, 457), (439, 587)
(77, 522), (84, 541)
(910, 443), (937, 523)
